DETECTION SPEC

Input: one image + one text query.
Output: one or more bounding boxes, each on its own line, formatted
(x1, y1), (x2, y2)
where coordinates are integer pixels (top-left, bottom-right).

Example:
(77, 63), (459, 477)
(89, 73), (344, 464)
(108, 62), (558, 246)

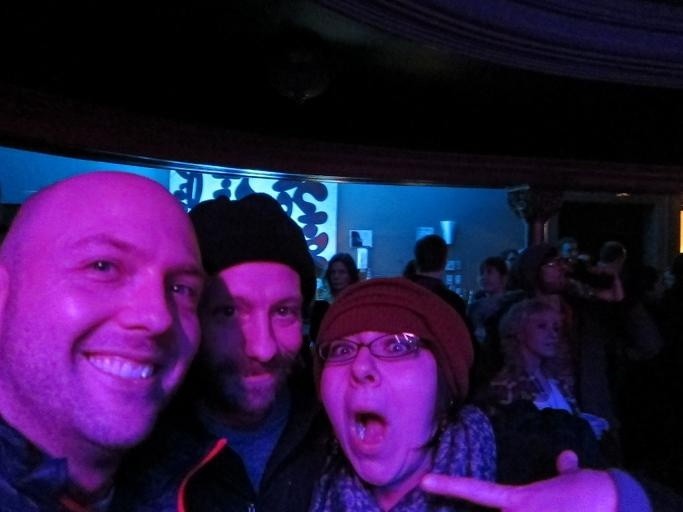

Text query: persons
(326, 252), (358, 295)
(411, 235), (467, 324)
(119, 189), (345, 512)
(0, 170), (210, 512)
(467, 237), (683, 483)
(276, 276), (680, 512)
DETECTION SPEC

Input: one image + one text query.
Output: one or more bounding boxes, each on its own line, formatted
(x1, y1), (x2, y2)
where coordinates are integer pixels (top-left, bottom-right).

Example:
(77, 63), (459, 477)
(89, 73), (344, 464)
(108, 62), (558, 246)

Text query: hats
(189, 192), (317, 306)
(313, 277), (475, 405)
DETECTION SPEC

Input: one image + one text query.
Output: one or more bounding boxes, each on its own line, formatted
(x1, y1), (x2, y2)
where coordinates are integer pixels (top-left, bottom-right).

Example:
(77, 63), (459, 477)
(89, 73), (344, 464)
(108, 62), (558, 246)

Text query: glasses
(318, 332), (420, 362)
